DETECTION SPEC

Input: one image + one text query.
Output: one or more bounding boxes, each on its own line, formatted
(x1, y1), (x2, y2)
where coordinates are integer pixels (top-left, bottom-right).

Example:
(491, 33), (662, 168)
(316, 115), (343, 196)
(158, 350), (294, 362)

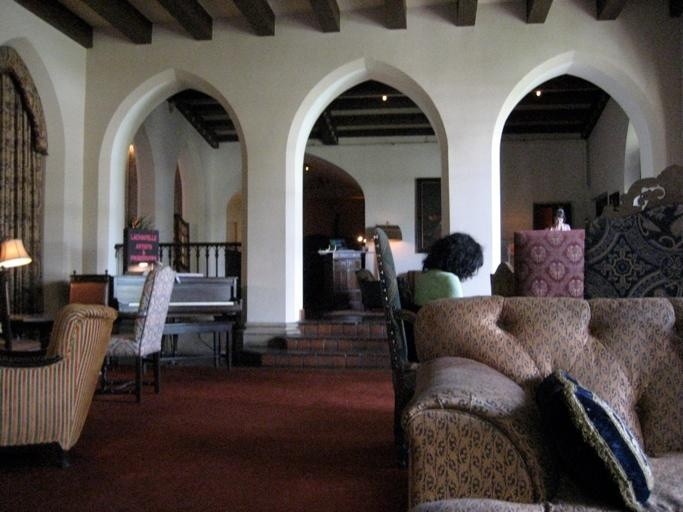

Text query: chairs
(97, 263), (178, 404)
(0, 273), (54, 361)
(1, 303), (117, 466)
(69, 274), (115, 308)
(374, 228), (423, 451)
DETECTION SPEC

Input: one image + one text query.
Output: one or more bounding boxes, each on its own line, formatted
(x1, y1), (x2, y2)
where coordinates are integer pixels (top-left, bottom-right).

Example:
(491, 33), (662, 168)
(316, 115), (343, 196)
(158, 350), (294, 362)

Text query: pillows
(539, 366), (655, 510)
(511, 227), (585, 300)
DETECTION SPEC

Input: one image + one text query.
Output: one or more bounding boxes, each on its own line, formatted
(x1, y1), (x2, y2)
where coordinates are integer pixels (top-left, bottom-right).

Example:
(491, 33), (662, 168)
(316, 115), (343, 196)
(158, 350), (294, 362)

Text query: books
(122, 227), (159, 276)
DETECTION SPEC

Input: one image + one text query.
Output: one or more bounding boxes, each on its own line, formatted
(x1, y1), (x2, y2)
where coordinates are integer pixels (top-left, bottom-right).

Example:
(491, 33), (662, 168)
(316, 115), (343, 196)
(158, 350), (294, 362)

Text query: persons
(413, 232), (484, 307)
(544, 208), (571, 232)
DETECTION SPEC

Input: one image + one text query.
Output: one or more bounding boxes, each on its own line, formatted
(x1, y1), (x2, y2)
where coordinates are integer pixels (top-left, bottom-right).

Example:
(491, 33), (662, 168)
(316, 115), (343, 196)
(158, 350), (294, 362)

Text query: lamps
(0, 237), (33, 269)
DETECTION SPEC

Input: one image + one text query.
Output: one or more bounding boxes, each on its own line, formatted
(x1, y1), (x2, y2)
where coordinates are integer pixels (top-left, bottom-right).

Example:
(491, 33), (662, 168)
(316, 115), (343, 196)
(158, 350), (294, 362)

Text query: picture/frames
(415, 176), (443, 253)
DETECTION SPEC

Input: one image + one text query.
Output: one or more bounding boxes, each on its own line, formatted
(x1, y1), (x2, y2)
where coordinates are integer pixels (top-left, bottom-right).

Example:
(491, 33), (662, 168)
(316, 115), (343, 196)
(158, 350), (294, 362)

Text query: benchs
(124, 320), (235, 377)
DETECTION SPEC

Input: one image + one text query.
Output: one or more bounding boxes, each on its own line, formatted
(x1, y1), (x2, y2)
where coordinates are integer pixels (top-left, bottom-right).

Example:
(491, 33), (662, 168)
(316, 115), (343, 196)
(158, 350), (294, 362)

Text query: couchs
(398, 294), (683, 512)
(488, 162), (683, 297)
